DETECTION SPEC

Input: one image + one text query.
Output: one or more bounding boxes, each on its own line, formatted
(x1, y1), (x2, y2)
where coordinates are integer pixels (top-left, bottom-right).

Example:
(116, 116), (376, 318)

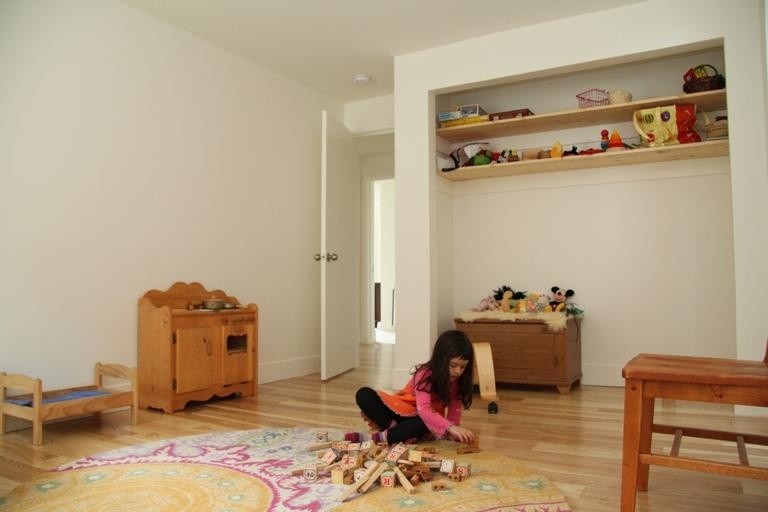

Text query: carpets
(0, 425), (573, 512)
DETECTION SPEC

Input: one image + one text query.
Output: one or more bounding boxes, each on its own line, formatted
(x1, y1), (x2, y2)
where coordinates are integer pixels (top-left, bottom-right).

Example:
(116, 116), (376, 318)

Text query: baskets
(683, 64), (725, 94)
(576, 90), (609, 108)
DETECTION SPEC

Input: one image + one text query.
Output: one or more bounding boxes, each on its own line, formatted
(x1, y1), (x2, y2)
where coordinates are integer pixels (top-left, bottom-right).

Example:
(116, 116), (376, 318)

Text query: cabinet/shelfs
(454, 313), (583, 395)
(136, 282), (259, 414)
(435, 89), (730, 183)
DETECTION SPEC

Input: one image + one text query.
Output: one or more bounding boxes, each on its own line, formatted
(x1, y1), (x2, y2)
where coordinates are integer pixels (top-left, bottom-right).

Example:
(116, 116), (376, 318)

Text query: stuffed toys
(471, 285), (574, 312)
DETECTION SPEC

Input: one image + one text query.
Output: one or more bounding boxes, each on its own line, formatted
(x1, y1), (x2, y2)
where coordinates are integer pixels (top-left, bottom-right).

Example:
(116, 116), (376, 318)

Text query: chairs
(620, 339), (768, 511)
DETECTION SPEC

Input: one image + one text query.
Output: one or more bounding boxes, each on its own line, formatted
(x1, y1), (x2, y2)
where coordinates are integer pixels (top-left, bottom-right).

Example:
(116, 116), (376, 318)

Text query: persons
(344, 328), (476, 445)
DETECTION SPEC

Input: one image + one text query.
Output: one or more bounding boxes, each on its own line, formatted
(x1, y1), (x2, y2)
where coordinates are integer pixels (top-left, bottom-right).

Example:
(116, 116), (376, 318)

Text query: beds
(0, 362), (139, 446)
